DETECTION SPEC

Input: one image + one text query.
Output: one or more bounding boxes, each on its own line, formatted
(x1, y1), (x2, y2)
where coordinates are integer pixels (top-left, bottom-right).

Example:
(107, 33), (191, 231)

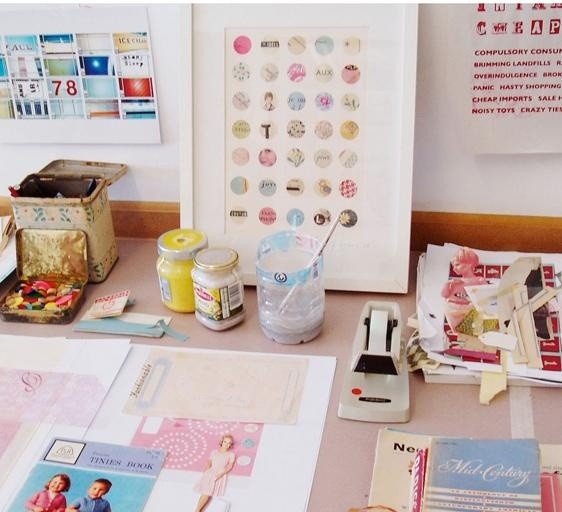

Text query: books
(367, 427), (561, 512)
(7, 437), (167, 512)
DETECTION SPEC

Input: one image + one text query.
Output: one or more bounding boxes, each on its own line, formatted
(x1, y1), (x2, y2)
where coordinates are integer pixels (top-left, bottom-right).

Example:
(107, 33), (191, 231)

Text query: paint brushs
(273, 211), (347, 318)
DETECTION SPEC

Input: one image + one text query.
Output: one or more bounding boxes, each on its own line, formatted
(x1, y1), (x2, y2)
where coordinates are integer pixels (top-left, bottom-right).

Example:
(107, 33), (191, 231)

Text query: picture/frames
(180, 3), (419, 295)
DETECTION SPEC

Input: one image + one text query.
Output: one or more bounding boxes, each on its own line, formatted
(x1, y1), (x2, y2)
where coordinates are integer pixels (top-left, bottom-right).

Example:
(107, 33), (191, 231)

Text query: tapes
(368, 309), (388, 354)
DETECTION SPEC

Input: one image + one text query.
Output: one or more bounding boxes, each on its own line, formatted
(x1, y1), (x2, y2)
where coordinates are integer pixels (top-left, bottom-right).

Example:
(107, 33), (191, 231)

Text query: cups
(255, 232), (327, 346)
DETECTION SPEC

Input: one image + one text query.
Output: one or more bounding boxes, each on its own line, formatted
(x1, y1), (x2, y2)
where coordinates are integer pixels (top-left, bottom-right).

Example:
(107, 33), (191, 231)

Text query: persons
(190, 433), (236, 512)
(26, 472), (71, 511)
(262, 92), (276, 111)
(64, 478), (114, 511)
(442, 248), (489, 334)
(259, 148), (276, 166)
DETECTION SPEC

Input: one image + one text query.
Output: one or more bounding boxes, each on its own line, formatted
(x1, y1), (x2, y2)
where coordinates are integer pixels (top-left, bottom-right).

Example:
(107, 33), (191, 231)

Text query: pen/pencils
(1, 215), (16, 251)
